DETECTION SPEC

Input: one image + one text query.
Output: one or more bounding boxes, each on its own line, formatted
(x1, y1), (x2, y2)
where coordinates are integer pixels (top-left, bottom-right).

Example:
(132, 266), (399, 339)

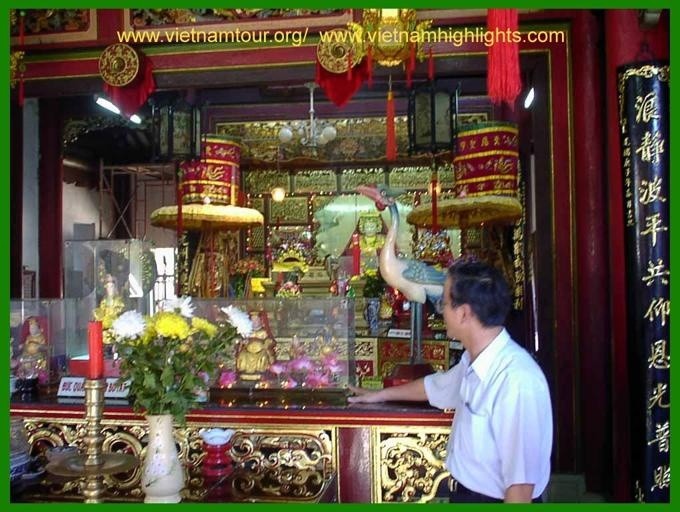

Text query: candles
(88, 318), (104, 380)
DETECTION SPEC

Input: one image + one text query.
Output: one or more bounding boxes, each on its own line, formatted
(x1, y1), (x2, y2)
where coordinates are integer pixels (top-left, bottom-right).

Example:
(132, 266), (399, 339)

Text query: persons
(237, 311), (274, 374)
(349, 212), (386, 258)
(93, 274), (124, 330)
(15, 318), (50, 376)
(346, 259), (554, 504)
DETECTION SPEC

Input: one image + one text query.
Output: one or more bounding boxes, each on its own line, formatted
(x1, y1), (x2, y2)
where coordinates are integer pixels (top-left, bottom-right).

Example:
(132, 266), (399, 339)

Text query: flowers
(95, 294), (252, 429)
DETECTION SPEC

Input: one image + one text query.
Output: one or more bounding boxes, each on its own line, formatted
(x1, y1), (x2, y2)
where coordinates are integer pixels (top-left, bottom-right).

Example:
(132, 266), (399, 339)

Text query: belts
(447, 475), (505, 504)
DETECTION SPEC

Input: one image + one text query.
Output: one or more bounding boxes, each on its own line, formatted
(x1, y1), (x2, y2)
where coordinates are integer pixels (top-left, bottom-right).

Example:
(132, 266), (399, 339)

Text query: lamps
(271, 156), (285, 204)
(94, 94), (143, 124)
(279, 82), (339, 159)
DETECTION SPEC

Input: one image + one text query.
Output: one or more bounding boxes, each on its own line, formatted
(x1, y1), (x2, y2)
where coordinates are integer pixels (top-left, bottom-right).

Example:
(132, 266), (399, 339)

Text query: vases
(141, 414), (183, 504)
